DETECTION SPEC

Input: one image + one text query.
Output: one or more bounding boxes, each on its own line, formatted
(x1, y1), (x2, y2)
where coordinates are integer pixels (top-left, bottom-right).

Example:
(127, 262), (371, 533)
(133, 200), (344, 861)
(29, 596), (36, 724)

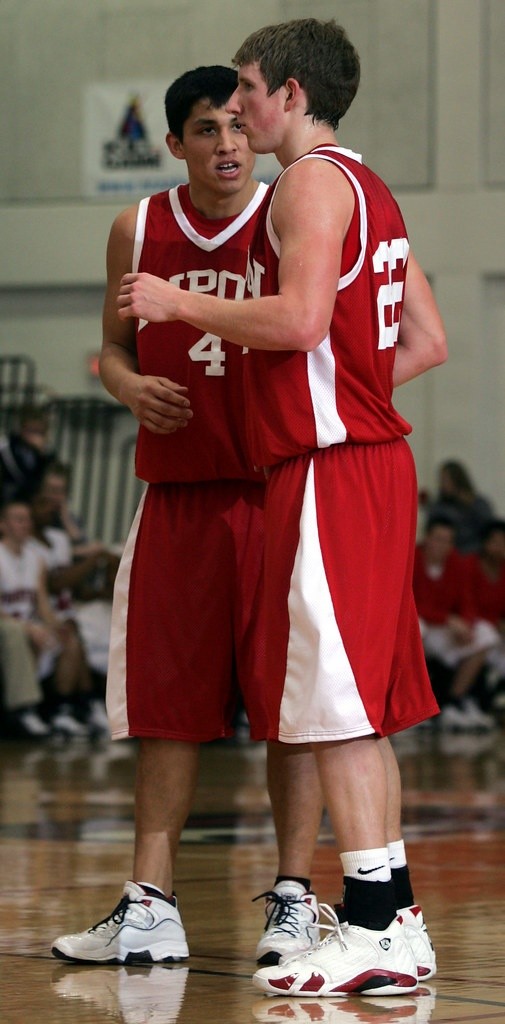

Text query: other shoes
(20, 710), (55, 738)
(437, 699), (495, 733)
(88, 701), (109, 729)
(52, 710), (97, 734)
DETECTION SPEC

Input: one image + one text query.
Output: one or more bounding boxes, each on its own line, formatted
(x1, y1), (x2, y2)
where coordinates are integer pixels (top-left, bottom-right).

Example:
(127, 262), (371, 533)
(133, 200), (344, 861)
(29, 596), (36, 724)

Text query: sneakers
(51, 880), (191, 966)
(249, 879), (321, 966)
(396, 902), (438, 982)
(249, 902), (419, 997)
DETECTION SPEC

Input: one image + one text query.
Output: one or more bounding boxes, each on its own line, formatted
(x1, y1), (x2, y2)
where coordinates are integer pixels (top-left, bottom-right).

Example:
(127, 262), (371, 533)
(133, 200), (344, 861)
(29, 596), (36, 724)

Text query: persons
(51, 63), (324, 964)
(409, 459), (505, 729)
(116, 18), (447, 997)
(0, 411), (127, 733)
